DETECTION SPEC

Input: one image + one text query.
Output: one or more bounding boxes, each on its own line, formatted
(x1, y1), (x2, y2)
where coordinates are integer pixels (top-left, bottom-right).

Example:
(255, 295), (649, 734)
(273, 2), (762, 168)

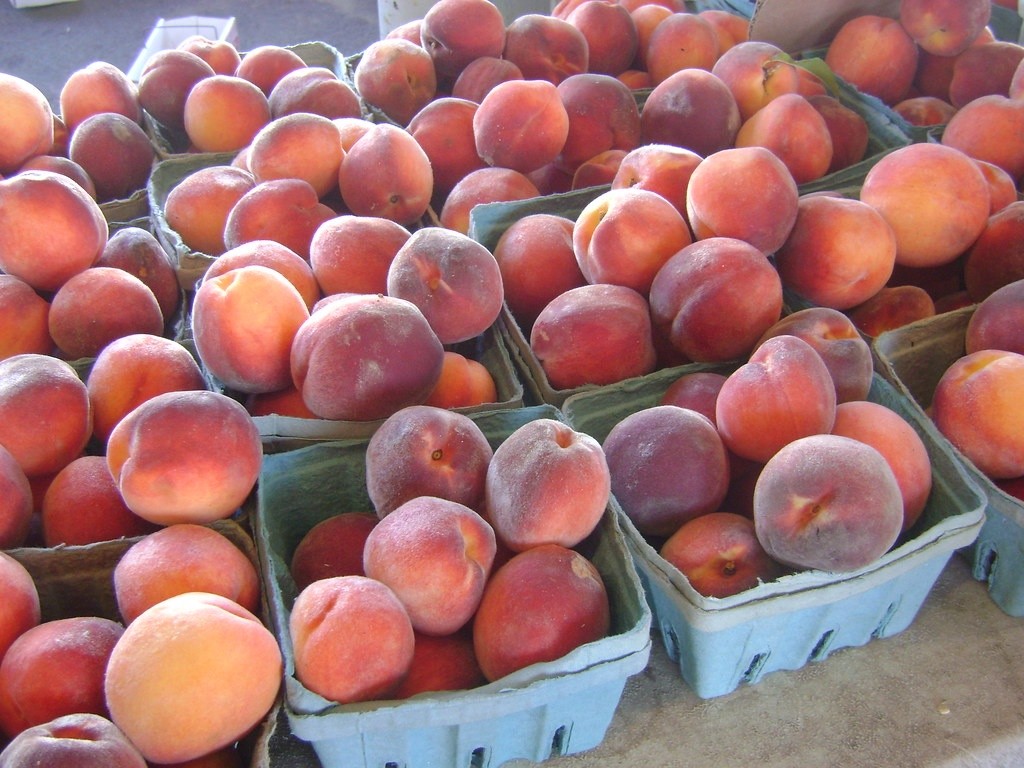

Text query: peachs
(0, 0), (1024, 768)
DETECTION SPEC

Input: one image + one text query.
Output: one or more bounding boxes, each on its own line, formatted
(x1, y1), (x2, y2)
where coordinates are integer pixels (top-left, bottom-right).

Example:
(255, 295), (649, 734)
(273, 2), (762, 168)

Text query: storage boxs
(1, 4), (1024, 766)
